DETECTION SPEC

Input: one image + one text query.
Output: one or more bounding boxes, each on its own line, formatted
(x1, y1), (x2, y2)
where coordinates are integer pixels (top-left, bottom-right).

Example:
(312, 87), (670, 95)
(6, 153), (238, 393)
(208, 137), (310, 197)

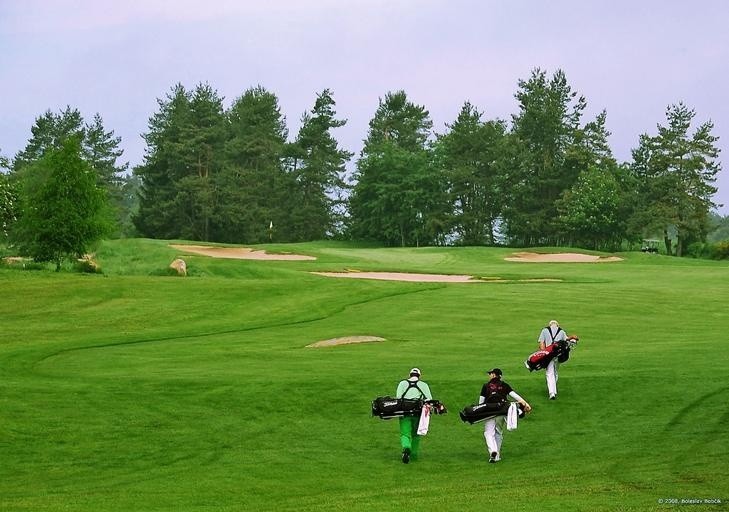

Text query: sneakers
(489, 451), (497, 463)
(549, 393), (556, 399)
(401, 448), (411, 463)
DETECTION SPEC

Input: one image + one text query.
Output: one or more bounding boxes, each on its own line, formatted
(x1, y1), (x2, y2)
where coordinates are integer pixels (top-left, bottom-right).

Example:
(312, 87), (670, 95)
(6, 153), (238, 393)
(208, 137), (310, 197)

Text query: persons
(396, 367), (433, 464)
(538, 320), (568, 400)
(477, 368), (532, 463)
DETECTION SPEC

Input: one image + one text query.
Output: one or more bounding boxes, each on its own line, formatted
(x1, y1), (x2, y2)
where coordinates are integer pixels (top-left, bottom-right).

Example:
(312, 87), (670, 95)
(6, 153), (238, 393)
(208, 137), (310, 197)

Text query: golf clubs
(565, 335), (579, 344)
(515, 401), (526, 418)
(423, 398), (448, 416)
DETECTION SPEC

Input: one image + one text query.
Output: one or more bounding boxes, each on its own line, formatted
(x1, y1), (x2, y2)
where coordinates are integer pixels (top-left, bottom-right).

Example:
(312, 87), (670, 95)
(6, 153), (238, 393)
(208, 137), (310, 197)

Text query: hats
(410, 367), (421, 374)
(487, 368), (502, 378)
(549, 320), (559, 326)
